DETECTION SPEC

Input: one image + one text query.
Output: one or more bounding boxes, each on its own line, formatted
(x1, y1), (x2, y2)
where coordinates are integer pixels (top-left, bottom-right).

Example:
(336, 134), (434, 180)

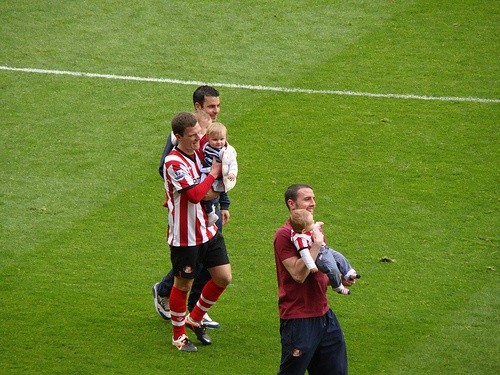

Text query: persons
(273, 183), (355, 375)
(152, 85), (230, 330)
(291, 208), (361, 296)
(163, 111), (232, 353)
(201, 122), (238, 227)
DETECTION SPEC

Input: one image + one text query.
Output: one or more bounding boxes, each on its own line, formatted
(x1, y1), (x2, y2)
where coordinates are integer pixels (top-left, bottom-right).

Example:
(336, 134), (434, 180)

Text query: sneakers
(170, 333), (198, 353)
(151, 283), (171, 321)
(185, 314), (211, 344)
(202, 313), (220, 328)
